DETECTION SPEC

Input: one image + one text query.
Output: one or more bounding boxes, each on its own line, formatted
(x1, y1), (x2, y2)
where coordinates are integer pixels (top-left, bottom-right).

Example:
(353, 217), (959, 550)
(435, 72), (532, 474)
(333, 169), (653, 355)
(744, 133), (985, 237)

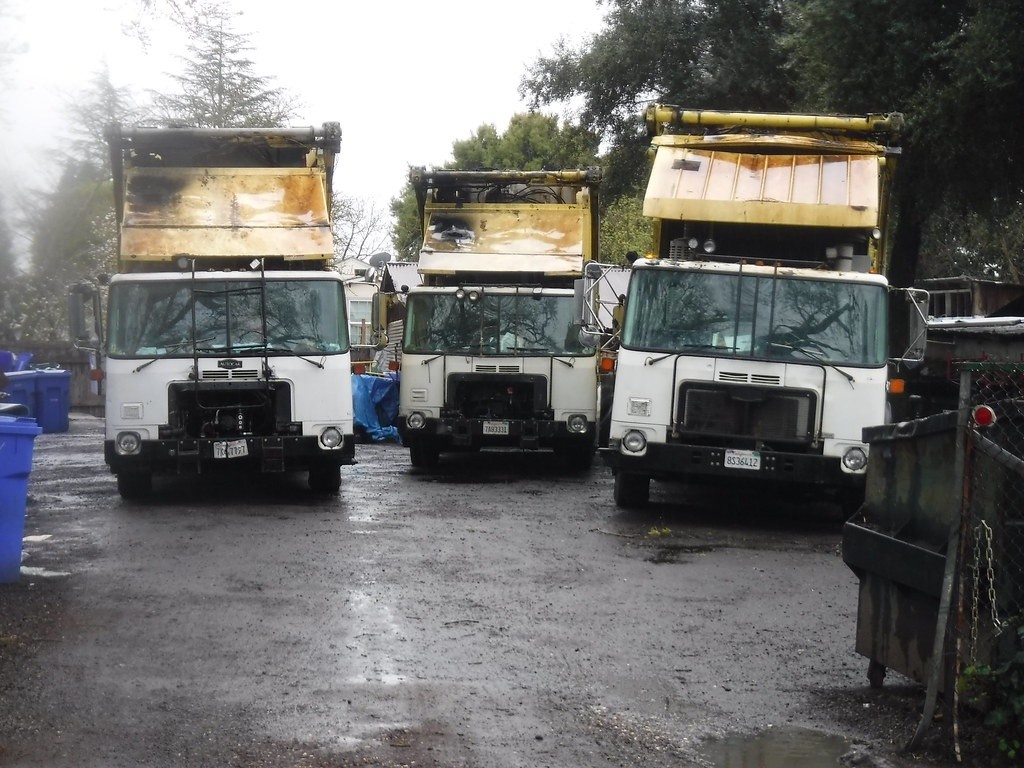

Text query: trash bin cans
(0, 350), (72, 433)
(0, 414), (43, 585)
(841, 397), (1024, 693)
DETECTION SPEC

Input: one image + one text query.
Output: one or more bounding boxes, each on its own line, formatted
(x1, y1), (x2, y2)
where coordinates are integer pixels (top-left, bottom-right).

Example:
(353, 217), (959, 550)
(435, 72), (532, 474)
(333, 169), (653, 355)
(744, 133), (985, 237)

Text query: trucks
(65, 119), (388, 502)
(366, 162), (628, 478)
(570, 103), (933, 515)
